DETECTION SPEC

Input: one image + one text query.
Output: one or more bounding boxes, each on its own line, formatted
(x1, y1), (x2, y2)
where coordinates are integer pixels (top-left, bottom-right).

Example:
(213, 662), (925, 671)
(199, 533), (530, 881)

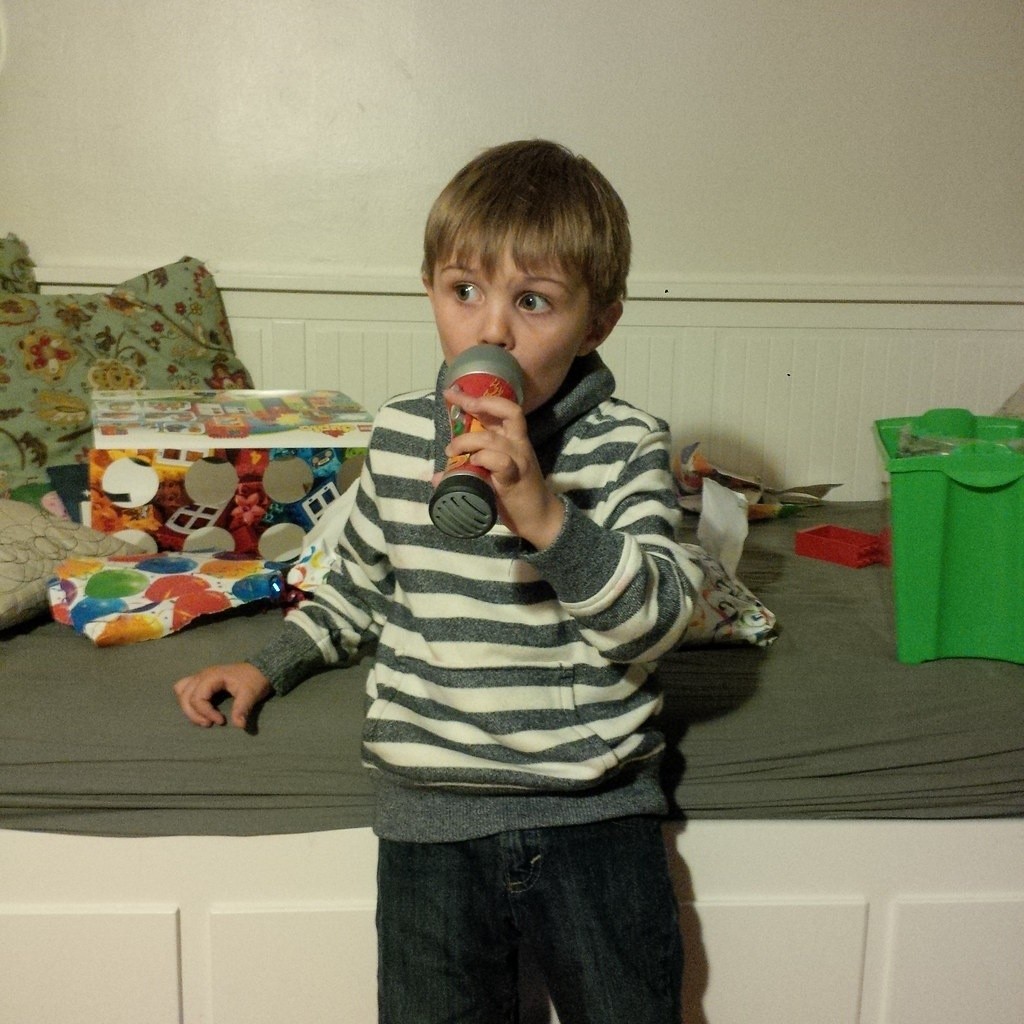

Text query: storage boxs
(873, 406), (1022, 669)
(795, 524), (879, 568)
(85, 390), (376, 565)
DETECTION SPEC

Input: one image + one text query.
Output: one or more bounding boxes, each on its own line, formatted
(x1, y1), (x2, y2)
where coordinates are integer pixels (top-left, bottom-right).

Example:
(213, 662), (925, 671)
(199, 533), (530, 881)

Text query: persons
(176, 137), (705, 1024)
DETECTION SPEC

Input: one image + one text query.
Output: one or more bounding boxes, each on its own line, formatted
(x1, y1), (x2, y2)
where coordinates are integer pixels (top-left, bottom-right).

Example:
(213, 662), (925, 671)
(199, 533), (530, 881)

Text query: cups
(428, 343), (526, 540)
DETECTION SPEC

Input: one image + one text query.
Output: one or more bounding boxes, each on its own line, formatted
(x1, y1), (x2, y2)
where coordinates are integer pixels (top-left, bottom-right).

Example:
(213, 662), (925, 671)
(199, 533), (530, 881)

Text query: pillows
(0, 499), (148, 643)
(1, 258), (254, 522)
